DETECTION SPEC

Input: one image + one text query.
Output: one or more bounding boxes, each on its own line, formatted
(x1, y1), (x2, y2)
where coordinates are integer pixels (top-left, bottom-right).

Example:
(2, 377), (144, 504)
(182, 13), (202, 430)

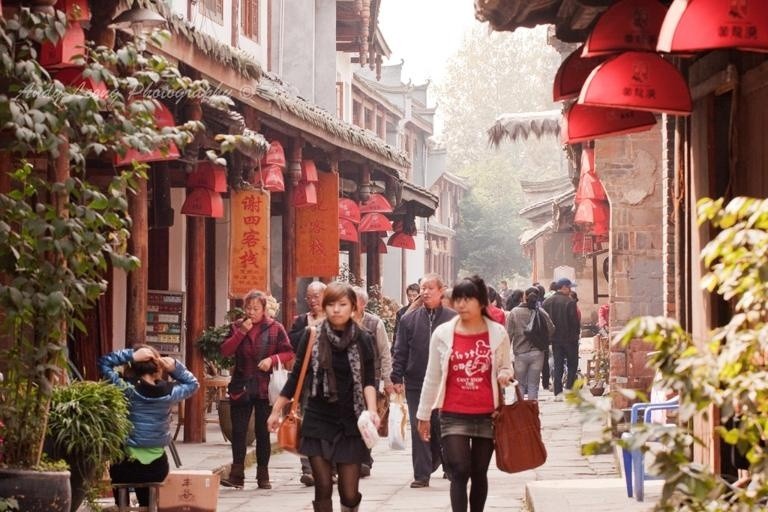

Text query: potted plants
(196, 306), (257, 446)
(589, 349), (605, 397)
(0, 0), (263, 512)
(16, 380), (135, 511)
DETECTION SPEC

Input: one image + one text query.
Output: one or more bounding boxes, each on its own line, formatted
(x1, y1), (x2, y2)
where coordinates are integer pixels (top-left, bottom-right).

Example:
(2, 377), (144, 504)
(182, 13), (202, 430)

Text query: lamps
(107, 0), (167, 52)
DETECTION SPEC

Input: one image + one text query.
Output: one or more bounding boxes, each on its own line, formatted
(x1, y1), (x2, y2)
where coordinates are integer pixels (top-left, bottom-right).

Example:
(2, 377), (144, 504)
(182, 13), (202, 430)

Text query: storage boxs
(157, 468), (221, 512)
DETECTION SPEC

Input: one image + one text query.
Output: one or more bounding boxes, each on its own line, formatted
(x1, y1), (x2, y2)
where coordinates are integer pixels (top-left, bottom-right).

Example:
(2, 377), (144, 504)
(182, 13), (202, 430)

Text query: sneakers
(300, 464), (371, 486)
(544, 383), (573, 401)
(410, 479), (429, 488)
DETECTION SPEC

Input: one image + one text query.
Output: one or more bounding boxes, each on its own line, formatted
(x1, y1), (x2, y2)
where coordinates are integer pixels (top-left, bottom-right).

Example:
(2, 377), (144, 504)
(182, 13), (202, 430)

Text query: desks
(167, 372), (238, 443)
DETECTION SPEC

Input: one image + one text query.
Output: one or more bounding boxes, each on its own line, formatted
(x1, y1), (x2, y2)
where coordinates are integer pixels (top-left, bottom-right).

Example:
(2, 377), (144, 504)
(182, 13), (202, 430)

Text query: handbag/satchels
(523, 309), (549, 351)
(375, 391), (409, 451)
(490, 377), (548, 474)
(267, 354), (295, 408)
(276, 414), (302, 455)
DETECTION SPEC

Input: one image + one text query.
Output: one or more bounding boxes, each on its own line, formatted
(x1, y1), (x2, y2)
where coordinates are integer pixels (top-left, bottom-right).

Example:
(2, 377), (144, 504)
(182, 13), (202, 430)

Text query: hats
(554, 278), (577, 289)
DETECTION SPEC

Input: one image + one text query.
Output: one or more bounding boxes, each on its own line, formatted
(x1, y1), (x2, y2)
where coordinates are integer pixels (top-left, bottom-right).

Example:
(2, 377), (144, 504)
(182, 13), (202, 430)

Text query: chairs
(619, 393), (679, 501)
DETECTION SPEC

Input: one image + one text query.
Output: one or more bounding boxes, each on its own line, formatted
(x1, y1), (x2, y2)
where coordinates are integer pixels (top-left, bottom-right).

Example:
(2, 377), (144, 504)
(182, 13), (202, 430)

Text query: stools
(110, 481), (164, 511)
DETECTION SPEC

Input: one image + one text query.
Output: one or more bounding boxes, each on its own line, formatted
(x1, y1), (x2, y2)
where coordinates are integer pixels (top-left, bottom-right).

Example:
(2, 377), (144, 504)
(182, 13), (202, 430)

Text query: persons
(95, 343), (201, 512)
(267, 281), (380, 512)
(219, 289), (294, 489)
(286, 280), (338, 486)
(352, 285), (394, 478)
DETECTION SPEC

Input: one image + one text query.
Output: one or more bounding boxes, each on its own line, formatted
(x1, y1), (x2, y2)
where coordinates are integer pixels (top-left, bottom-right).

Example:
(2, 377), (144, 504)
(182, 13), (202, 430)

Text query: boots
(312, 492), (362, 512)
(219, 463), (245, 489)
(255, 464), (272, 490)
